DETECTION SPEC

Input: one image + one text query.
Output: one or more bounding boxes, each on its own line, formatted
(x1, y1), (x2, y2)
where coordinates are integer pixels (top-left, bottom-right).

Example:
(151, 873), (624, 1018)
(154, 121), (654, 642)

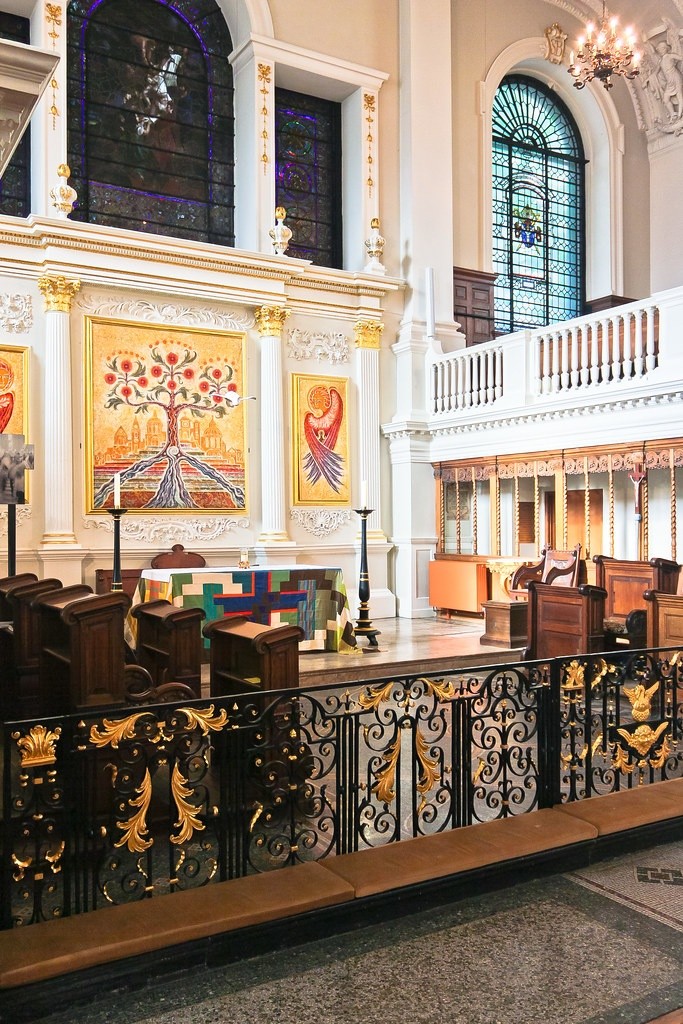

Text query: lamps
(568, 0), (641, 91)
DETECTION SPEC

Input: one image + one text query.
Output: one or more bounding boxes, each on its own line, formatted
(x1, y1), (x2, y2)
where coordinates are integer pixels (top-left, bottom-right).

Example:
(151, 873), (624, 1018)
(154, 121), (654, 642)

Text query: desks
(124, 564), (365, 654)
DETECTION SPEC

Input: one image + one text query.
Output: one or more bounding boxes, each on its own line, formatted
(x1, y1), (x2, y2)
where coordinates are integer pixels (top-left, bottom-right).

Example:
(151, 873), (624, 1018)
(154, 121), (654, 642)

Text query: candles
(113, 472), (121, 509)
(362, 480), (368, 509)
(241, 549), (248, 562)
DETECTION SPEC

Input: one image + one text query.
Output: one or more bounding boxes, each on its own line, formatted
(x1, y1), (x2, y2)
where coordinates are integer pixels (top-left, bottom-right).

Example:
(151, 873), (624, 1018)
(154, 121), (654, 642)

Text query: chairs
(509, 545), (584, 602)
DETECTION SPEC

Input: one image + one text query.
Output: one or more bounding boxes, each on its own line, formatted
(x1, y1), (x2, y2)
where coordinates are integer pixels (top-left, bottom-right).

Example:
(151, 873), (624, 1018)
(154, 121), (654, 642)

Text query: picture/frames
(292, 371), (351, 507)
(85, 315), (249, 514)
(0, 344), (31, 505)
